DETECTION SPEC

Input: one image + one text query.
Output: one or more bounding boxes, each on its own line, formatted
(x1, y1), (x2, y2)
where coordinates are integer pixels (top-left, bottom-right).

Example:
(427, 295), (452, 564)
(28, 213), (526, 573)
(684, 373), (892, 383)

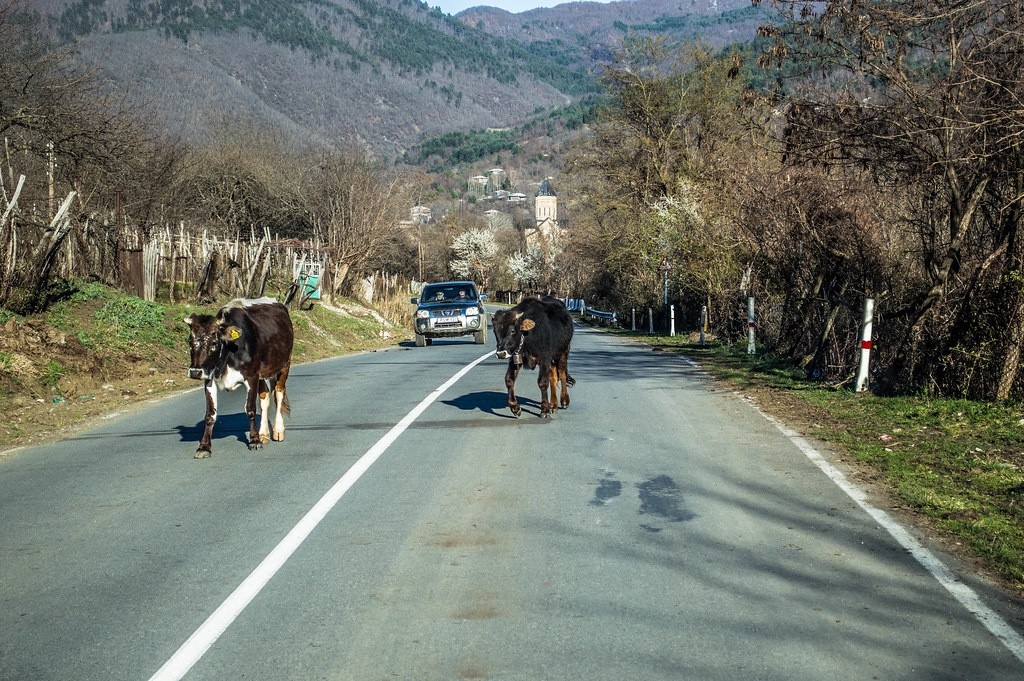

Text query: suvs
(411, 281), (488, 347)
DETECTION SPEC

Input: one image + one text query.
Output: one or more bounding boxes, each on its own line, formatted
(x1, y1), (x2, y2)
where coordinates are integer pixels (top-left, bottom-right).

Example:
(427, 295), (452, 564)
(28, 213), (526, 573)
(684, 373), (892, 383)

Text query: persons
(427, 290), (448, 302)
(456, 286), (470, 299)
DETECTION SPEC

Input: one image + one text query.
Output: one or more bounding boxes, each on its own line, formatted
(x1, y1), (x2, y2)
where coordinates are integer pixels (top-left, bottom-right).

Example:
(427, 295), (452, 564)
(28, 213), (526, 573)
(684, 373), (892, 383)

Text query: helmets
(458, 287), (466, 293)
(435, 290), (444, 300)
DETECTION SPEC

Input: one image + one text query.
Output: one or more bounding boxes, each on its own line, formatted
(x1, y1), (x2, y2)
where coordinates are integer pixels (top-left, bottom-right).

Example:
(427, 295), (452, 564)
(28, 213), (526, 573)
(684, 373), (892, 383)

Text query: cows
(183, 295), (294, 459)
(489, 295), (576, 418)
